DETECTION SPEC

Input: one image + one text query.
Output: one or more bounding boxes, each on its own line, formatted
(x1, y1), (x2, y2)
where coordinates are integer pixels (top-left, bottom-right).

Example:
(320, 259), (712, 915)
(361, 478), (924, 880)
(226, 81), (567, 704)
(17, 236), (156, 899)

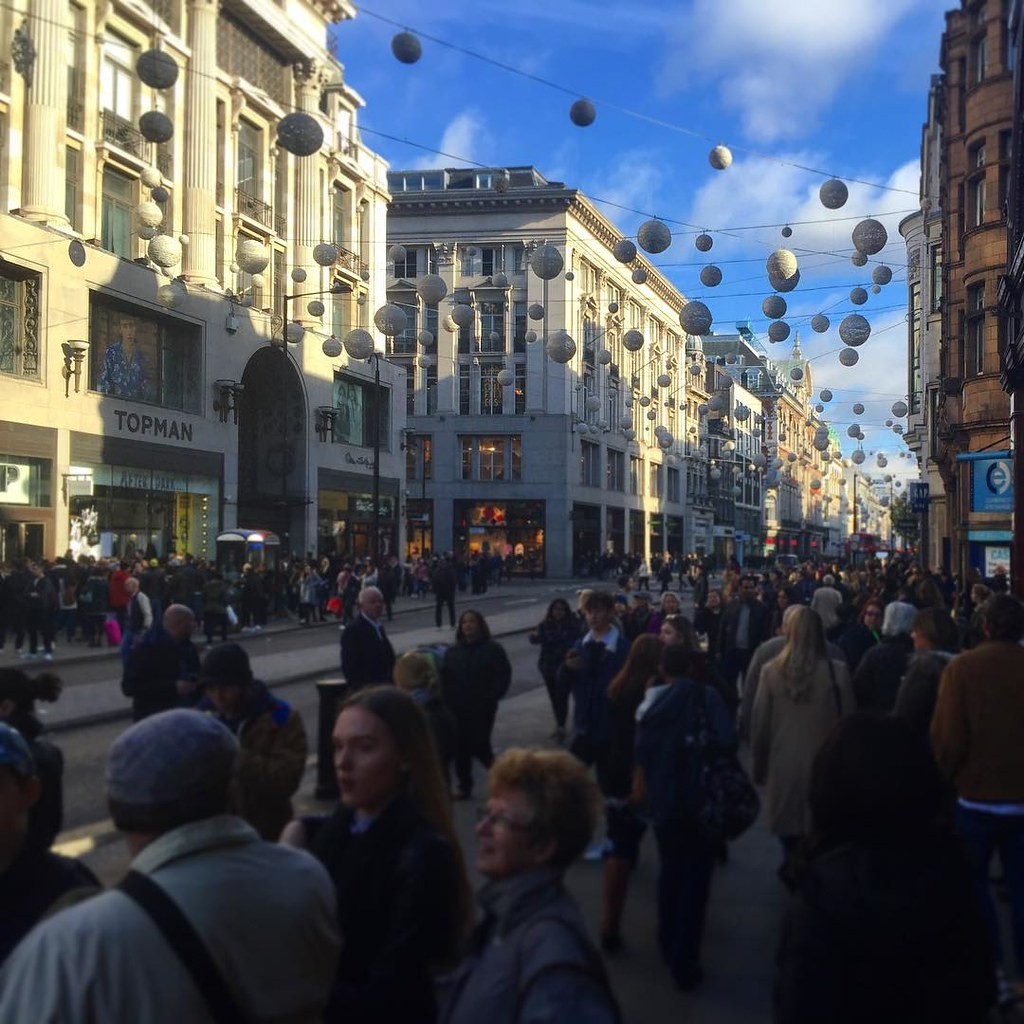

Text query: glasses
(866, 611), (882, 617)
(476, 806), (538, 833)
(665, 614), (679, 622)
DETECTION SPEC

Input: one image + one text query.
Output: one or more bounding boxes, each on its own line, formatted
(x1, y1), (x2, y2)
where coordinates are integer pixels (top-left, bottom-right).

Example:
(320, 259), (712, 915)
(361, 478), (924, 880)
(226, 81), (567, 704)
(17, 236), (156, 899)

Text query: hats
(0, 722), (32, 785)
(196, 643), (250, 687)
(104, 708), (235, 804)
(615, 593), (627, 604)
(634, 591), (652, 603)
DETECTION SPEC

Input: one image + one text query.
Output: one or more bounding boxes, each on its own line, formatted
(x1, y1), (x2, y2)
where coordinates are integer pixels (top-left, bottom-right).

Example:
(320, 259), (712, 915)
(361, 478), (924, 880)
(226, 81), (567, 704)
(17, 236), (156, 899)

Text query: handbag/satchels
(226, 607), (239, 625)
(699, 761), (760, 839)
(79, 591), (93, 604)
(326, 598), (342, 614)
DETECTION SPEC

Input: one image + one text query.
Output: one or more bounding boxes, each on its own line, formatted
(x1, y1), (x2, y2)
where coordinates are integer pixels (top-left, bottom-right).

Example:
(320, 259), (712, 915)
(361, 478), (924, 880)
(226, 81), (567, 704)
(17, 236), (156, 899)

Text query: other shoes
(453, 784), (473, 801)
(601, 931), (621, 951)
(657, 934), (702, 992)
(585, 839), (614, 861)
(556, 725), (565, 736)
(15, 641), (55, 661)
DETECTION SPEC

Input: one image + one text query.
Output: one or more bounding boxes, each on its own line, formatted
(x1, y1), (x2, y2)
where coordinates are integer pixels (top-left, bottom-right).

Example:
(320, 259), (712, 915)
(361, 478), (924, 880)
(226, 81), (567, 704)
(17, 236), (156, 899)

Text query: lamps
(214, 379), (244, 425)
(155, 503), (165, 514)
(62, 340), (91, 393)
(315, 406), (340, 443)
(401, 428), (416, 451)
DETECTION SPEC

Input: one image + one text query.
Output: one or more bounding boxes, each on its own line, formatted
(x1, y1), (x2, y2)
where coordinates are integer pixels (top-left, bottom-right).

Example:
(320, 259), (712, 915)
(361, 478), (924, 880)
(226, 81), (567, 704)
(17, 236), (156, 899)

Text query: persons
(279, 683), (473, 1024)
(530, 556), (1024, 1024)
(588, 549), (717, 594)
(0, 666), (103, 961)
(0, 546), (515, 660)
(338, 586), (512, 801)
(0, 709), (336, 1024)
(121, 603), (203, 723)
(434, 748), (624, 1024)
(199, 642), (307, 840)
